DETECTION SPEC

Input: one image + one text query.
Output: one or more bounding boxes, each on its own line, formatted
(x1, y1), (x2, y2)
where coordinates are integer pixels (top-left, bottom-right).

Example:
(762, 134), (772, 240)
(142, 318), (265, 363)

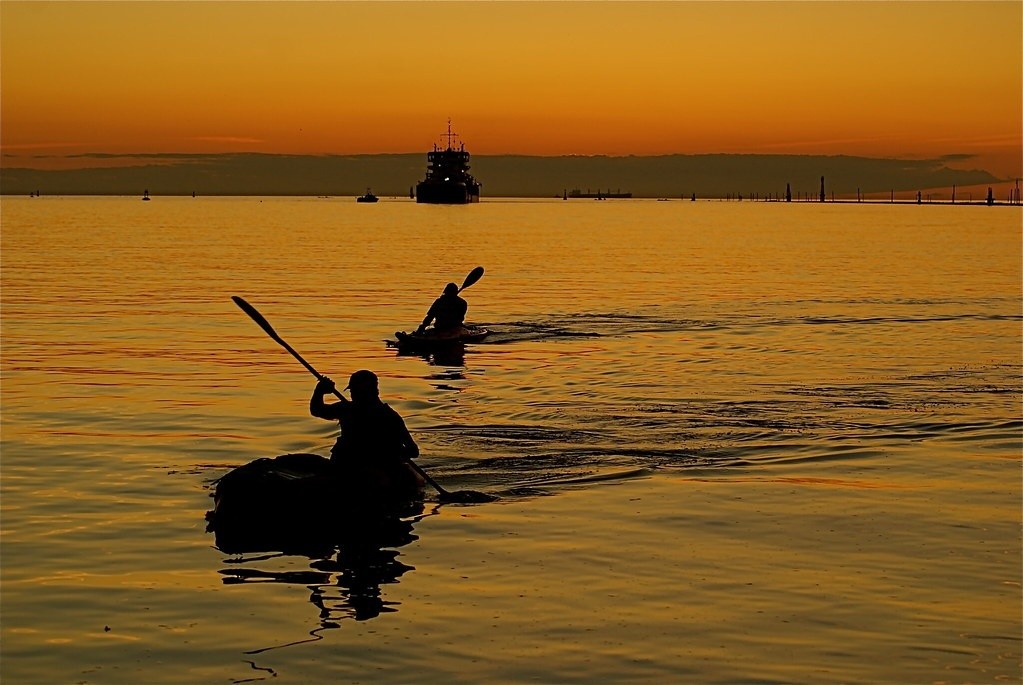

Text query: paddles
(229, 293), (492, 501)
(458, 266), (484, 296)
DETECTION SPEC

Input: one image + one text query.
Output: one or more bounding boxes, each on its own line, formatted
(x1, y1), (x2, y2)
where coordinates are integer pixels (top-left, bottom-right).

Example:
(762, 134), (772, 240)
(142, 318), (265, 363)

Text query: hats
(343, 371), (378, 391)
(443, 282), (459, 293)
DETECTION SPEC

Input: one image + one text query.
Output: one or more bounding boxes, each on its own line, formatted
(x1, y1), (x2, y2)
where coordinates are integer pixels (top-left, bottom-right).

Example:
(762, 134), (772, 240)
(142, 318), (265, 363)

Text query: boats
(416, 120), (481, 204)
(395, 324), (488, 346)
(205, 454), (425, 533)
(357, 192), (379, 203)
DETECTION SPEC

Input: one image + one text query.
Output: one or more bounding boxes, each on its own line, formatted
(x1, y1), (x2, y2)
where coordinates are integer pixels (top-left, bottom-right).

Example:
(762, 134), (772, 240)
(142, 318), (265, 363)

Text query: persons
(418, 283), (467, 334)
(310, 371), (418, 471)
